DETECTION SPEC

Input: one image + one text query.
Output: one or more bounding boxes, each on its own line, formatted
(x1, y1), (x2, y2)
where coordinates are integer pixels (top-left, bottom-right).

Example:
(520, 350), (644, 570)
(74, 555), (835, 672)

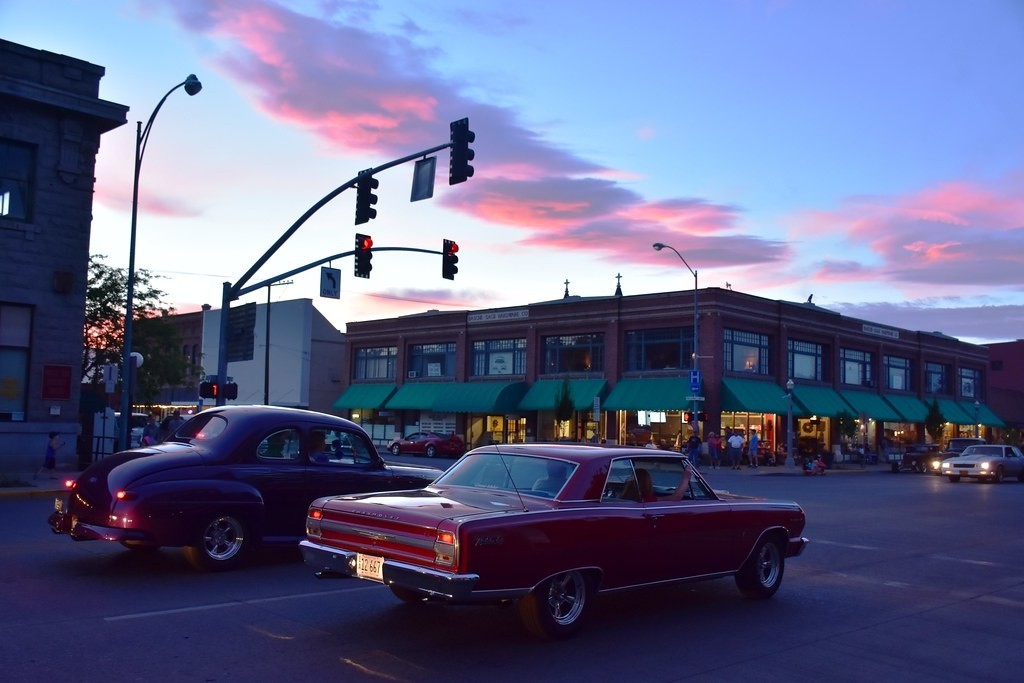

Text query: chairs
(622, 466), (652, 502)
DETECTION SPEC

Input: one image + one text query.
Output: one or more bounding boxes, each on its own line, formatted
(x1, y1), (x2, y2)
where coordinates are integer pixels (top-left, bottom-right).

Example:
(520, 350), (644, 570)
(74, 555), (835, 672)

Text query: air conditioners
(408, 370), (419, 379)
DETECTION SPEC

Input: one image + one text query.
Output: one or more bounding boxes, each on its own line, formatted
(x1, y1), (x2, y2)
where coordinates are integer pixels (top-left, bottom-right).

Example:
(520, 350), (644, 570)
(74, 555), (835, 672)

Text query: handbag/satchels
(690, 438), (699, 448)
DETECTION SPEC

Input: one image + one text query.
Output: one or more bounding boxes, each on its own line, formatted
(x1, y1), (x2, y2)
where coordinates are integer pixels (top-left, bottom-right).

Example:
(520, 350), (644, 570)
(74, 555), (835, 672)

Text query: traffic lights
(356, 167), (378, 224)
(355, 234), (372, 278)
(443, 239), (458, 280)
(200, 382), (218, 399)
(449, 117), (475, 185)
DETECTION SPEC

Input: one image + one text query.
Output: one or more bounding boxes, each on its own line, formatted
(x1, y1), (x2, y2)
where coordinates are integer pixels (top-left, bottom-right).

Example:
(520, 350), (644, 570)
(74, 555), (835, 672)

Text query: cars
(46, 403), (434, 572)
(892, 443), (943, 474)
(387, 431), (466, 459)
(941, 445), (1024, 483)
(930, 438), (986, 476)
(298, 443), (808, 643)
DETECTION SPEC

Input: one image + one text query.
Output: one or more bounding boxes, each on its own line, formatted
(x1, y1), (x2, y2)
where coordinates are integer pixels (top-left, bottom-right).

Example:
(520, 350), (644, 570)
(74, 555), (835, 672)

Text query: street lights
(784, 380), (795, 467)
(654, 244), (699, 438)
(974, 402), (980, 438)
(118, 75), (203, 449)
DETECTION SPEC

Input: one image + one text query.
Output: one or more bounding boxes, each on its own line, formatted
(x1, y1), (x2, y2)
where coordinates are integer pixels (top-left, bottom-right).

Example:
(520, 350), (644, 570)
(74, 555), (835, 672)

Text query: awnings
(332, 380), (529, 414)
(521, 377), (704, 411)
(721, 376), (1007, 428)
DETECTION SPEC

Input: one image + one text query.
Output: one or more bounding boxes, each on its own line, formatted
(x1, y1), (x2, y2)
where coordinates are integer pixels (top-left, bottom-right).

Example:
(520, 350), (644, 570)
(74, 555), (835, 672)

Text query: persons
(309, 431), (328, 463)
(847, 436), (901, 464)
(35, 432), (68, 480)
(776, 442), (827, 477)
(265, 432), (286, 457)
(553, 429), (599, 443)
(646, 424), (758, 470)
(140, 411), (187, 447)
(621, 465), (693, 501)
(530, 459), (568, 496)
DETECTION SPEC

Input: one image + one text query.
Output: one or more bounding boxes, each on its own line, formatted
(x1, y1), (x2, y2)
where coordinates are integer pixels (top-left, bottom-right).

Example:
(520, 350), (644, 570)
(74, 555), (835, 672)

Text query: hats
(48, 431), (60, 439)
(144, 415), (156, 421)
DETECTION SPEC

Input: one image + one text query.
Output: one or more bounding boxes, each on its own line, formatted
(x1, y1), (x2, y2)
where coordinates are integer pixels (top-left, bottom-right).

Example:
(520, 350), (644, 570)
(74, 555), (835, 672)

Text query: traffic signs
(320, 267), (340, 299)
